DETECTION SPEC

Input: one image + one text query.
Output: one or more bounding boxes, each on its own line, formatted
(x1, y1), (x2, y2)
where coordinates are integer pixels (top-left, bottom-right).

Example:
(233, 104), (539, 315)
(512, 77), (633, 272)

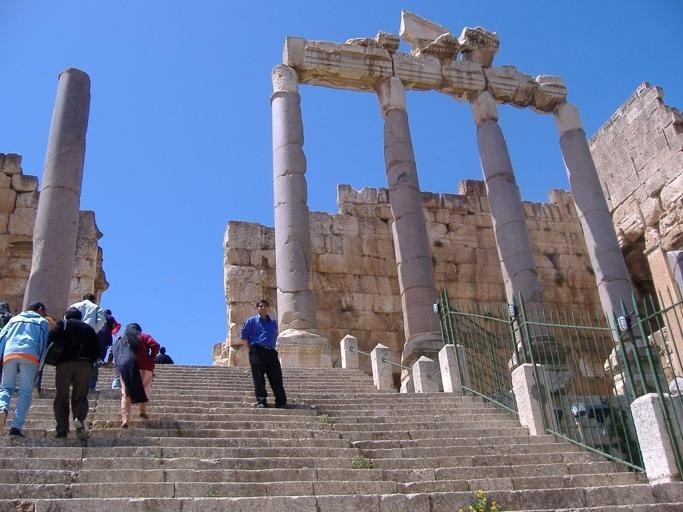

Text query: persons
(0, 290), (173, 439)
(240, 300), (292, 409)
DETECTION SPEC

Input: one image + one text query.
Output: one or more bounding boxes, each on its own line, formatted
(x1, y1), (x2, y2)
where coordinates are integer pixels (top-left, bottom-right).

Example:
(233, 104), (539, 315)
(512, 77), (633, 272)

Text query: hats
(30, 301), (48, 316)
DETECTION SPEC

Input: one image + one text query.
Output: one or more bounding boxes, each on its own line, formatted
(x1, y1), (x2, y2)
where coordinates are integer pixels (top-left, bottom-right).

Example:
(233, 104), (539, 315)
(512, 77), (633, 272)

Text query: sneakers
(73, 417), (86, 439)
(257, 400), (267, 409)
(139, 413), (150, 420)
(55, 433), (68, 438)
(0, 410), (7, 429)
(275, 401), (287, 408)
(121, 422), (128, 428)
(9, 427), (26, 438)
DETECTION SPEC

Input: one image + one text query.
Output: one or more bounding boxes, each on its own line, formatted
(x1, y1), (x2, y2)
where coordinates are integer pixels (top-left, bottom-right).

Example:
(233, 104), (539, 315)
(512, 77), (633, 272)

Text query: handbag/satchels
(44, 337), (64, 366)
(112, 323), (121, 335)
(112, 376), (120, 389)
